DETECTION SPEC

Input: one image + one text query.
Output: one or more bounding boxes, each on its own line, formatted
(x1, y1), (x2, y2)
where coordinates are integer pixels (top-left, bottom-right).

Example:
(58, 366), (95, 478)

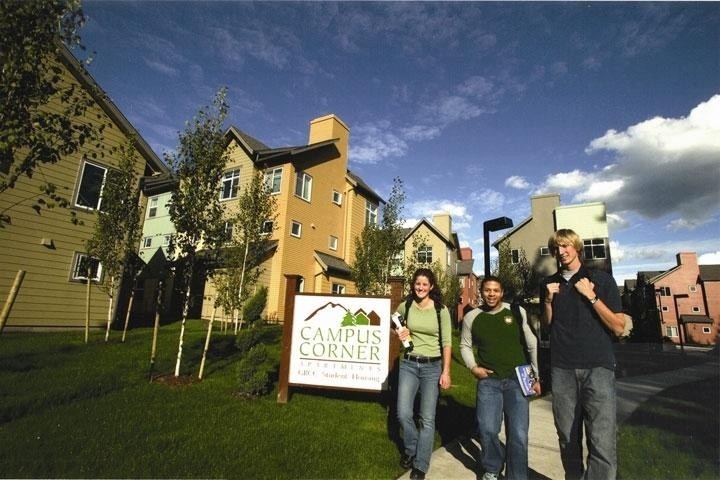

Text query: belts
(404, 353), (441, 364)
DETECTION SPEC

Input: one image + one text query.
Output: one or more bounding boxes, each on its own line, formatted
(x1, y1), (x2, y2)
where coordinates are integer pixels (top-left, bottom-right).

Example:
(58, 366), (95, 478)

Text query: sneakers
(400, 453), (415, 469)
(410, 468), (425, 480)
(481, 471), (501, 480)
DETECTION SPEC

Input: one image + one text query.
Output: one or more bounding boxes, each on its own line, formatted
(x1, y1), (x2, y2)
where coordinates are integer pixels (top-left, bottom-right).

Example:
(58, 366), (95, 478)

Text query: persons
(460, 277), (547, 480)
(391, 268), (452, 479)
(540, 229), (626, 479)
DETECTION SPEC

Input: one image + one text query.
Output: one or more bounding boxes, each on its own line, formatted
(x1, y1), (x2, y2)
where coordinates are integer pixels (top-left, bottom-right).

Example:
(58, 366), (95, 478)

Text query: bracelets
(544, 296), (552, 304)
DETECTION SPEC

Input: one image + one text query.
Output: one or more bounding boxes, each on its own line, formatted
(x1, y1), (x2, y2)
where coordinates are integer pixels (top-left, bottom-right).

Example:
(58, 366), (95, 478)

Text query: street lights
(483, 216), (514, 277)
(673, 294), (691, 350)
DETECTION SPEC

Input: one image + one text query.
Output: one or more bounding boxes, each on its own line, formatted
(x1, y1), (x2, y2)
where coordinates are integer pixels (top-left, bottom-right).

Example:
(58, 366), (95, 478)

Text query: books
(515, 364), (537, 396)
(392, 315), (412, 347)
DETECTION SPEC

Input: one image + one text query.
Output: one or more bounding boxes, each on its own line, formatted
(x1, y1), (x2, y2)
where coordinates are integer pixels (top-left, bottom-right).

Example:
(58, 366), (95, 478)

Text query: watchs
(589, 294), (598, 304)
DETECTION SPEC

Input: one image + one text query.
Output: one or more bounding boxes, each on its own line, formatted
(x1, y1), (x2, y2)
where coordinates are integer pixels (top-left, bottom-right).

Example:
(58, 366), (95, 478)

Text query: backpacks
(510, 302), (543, 371)
